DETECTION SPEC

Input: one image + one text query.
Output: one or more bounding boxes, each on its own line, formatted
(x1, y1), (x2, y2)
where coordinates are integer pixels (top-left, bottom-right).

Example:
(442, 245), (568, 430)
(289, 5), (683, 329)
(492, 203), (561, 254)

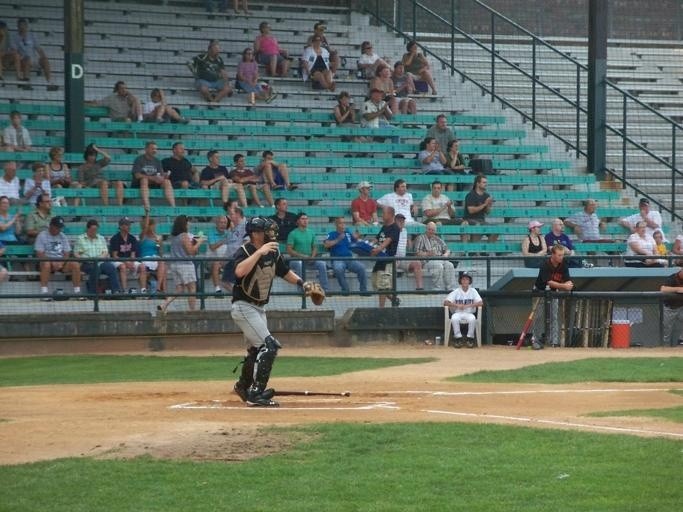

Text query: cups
(435, 337), (440, 344)
(507, 341), (511, 347)
(193, 237), (199, 248)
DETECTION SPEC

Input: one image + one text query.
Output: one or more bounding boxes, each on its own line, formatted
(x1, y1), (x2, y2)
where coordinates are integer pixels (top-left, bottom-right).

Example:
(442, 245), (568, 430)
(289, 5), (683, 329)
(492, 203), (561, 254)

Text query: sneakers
(140, 287), (150, 300)
(156, 304), (166, 312)
(466, 338), (475, 349)
(40, 293), (51, 301)
(454, 338), (464, 349)
(74, 292), (87, 301)
(215, 286), (221, 293)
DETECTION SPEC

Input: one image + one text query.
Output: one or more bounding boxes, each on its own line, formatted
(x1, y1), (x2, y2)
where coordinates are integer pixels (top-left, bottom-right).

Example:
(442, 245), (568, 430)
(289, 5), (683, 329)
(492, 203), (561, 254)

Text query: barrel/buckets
(611, 319), (633, 348)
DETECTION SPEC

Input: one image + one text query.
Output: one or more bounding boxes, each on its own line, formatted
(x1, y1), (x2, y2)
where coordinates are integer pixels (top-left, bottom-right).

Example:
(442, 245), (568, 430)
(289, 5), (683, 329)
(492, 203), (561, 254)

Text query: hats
(527, 220), (545, 230)
(50, 216), (65, 228)
(369, 87), (385, 95)
(357, 180), (373, 191)
(639, 197), (650, 206)
(118, 216), (135, 226)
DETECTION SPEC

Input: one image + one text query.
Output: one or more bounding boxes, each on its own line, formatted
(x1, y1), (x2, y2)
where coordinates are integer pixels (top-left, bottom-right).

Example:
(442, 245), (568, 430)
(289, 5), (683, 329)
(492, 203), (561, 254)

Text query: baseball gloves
(303, 281), (326, 305)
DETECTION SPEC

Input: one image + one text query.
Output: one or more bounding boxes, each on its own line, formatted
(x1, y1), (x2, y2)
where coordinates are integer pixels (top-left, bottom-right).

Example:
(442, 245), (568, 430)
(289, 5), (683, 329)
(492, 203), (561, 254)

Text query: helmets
(458, 272), (473, 285)
(245, 216), (279, 236)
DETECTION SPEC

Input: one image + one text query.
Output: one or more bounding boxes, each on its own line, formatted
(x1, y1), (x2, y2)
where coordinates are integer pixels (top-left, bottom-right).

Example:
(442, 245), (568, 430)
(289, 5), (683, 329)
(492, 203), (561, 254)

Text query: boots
(234, 381), (275, 402)
(245, 381), (279, 408)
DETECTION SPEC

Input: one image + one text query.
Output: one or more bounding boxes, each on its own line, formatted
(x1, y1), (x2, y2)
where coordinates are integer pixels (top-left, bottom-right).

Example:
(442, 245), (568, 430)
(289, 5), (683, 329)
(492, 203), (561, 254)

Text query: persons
(444, 272), (483, 348)
(1, 18), (367, 307)
(327, 40), (438, 158)
(530, 246), (573, 350)
(222, 218), (325, 406)
(661, 268), (683, 346)
(346, 116), (682, 308)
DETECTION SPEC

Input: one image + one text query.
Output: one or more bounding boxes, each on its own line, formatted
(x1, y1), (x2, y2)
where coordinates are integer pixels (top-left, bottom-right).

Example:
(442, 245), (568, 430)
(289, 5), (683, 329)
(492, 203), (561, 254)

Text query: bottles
(150, 281), (156, 296)
(250, 92), (255, 104)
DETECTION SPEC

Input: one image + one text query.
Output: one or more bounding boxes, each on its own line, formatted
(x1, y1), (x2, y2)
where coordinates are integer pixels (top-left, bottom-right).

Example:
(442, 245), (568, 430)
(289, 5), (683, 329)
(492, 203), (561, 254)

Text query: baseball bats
(516, 298), (540, 350)
(274, 390), (350, 397)
(560, 298), (612, 347)
